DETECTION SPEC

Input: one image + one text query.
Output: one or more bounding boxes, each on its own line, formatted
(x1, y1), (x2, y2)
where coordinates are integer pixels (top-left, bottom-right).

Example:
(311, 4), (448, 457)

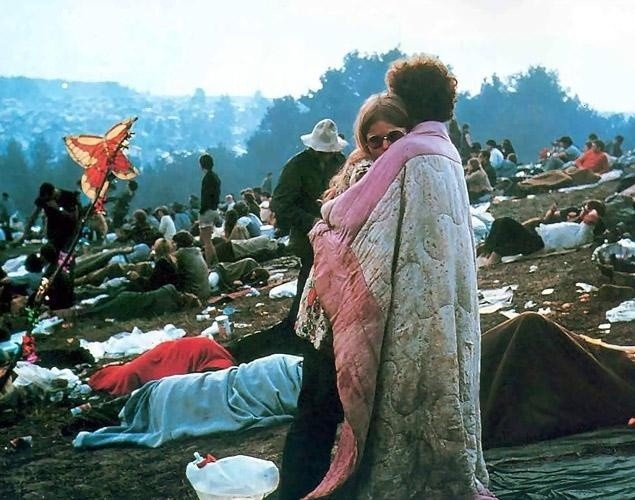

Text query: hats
(300, 119), (347, 152)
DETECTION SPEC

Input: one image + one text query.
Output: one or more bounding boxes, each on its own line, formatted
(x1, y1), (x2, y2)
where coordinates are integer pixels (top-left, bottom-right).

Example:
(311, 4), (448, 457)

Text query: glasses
(365, 130), (409, 149)
